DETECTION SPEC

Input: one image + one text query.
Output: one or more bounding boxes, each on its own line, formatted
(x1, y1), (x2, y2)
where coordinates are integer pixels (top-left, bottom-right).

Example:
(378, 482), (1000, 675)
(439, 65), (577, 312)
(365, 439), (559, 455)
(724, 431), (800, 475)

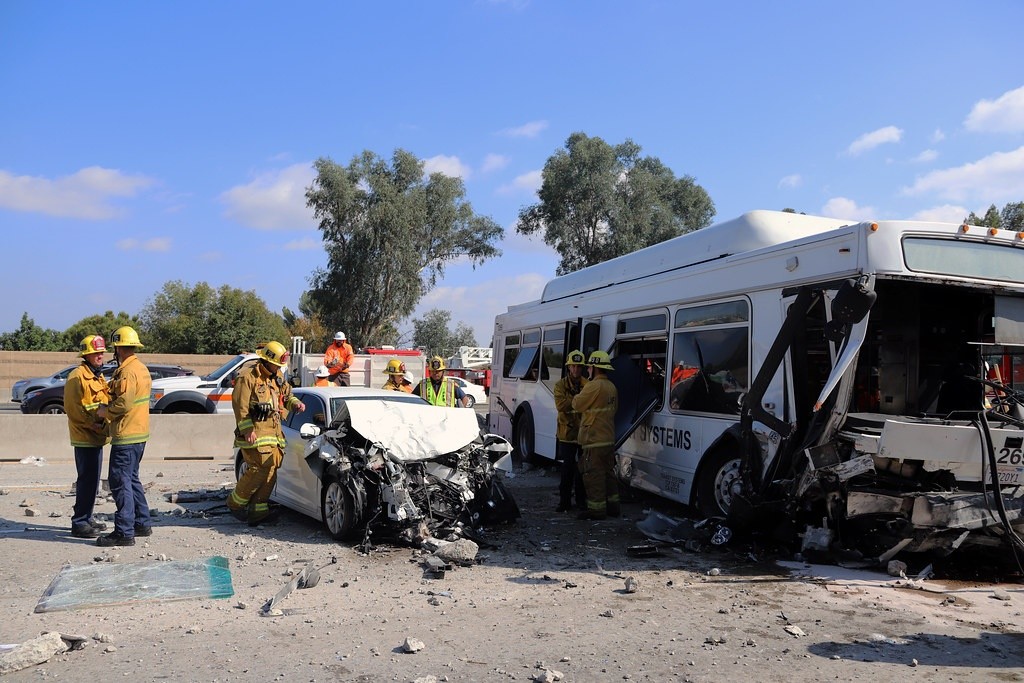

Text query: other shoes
(576, 508), (606, 521)
(608, 504), (620, 518)
(71, 525), (101, 536)
(134, 522), (152, 536)
(225, 500), (249, 522)
(573, 503), (587, 510)
(89, 519), (107, 531)
(249, 509), (278, 527)
(96, 531), (136, 547)
(555, 502), (571, 512)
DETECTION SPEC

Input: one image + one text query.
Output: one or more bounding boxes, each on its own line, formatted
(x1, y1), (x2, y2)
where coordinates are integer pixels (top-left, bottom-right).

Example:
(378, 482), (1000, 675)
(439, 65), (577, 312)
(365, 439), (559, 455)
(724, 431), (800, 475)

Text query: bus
(487, 209), (1024, 569)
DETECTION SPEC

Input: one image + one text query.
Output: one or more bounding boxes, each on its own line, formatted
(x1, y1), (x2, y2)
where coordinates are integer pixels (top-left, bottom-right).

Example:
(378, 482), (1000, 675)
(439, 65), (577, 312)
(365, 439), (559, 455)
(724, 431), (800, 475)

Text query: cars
(235, 386), (521, 547)
(444, 376), (488, 408)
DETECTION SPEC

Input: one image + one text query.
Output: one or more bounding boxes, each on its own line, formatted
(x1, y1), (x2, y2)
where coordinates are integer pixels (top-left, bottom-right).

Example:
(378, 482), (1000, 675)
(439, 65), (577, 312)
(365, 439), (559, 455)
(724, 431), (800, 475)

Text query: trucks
(149, 353), (428, 415)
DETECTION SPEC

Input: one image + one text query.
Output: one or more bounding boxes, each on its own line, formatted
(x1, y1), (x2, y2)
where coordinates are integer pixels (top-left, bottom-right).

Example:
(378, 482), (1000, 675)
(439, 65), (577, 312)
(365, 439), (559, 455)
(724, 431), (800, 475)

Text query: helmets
(255, 341), (289, 367)
(314, 366), (330, 377)
(565, 350), (586, 366)
(584, 351), (616, 372)
(427, 356), (445, 371)
(333, 331), (346, 341)
(76, 335), (107, 358)
(108, 326), (144, 348)
(382, 359), (405, 375)
(403, 371), (413, 385)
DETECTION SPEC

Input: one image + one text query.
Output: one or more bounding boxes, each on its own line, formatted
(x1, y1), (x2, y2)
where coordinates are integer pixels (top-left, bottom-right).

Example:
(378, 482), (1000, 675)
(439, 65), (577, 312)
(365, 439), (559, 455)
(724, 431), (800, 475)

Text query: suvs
(11, 362), (195, 414)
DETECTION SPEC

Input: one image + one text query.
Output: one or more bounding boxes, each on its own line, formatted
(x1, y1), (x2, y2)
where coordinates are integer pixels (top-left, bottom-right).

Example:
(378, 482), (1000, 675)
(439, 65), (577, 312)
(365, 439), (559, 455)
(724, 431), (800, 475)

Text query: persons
(382, 359), (407, 392)
(96, 325), (153, 546)
(225, 341), (305, 527)
(672, 360), (699, 385)
(308, 365), (337, 388)
(324, 331), (354, 387)
(64, 334), (112, 537)
(400, 371), (414, 392)
(570, 350), (621, 520)
(554, 349), (590, 512)
(411, 355), (468, 408)
